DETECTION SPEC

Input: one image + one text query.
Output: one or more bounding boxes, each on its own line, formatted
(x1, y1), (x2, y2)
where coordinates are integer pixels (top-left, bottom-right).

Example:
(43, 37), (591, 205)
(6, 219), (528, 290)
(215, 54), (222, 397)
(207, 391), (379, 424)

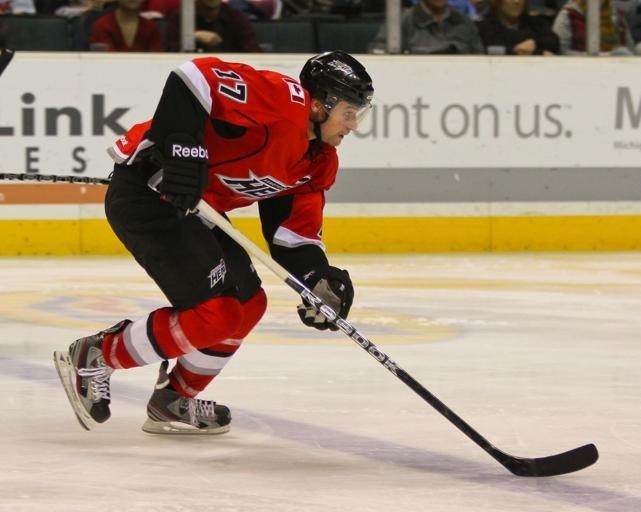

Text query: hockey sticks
(140, 155), (599, 477)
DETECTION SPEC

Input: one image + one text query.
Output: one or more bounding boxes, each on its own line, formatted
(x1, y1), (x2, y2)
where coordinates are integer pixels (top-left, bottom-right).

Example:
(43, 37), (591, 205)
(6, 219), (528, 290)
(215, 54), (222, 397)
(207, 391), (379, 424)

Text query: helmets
(298, 50), (375, 112)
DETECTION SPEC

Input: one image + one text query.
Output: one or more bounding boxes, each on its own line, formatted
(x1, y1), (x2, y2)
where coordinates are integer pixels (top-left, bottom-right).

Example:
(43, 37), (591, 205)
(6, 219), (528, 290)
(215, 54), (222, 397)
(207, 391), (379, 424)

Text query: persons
(67, 49), (374, 431)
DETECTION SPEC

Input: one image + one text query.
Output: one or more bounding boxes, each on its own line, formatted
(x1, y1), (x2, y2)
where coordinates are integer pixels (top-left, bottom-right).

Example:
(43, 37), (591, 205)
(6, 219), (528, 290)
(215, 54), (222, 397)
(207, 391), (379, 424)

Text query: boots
(146, 359), (233, 432)
(67, 314), (134, 425)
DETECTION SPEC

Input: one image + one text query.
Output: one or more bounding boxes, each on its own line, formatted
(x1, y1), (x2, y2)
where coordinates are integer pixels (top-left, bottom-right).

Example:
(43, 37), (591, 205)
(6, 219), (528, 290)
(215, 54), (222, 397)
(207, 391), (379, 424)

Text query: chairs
(250, 19), (385, 52)
(474, 15), (553, 44)
(2, 13), (91, 51)
(153, 18), (179, 46)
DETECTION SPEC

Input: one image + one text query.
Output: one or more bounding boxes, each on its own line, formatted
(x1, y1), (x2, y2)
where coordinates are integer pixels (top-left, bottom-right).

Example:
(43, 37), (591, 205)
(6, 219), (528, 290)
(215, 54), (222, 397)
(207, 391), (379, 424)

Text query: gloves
(295, 260), (358, 333)
(154, 134), (212, 216)
